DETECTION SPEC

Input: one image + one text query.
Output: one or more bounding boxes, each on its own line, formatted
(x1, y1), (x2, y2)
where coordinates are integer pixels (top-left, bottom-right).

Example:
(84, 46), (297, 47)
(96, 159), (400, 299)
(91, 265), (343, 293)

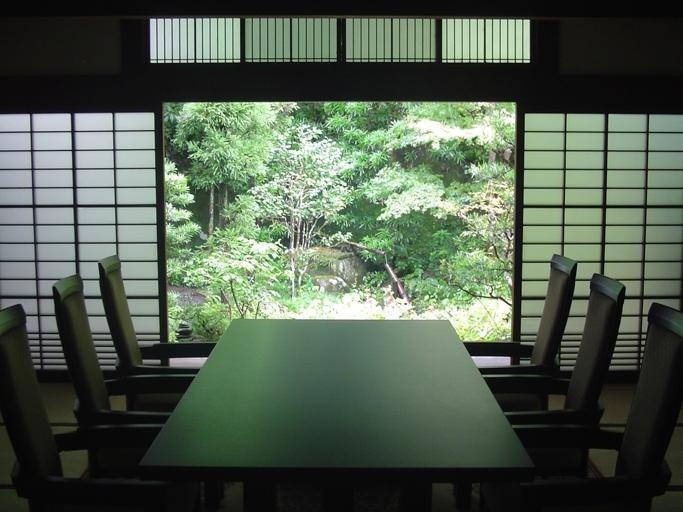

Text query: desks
(136, 318), (537, 510)
